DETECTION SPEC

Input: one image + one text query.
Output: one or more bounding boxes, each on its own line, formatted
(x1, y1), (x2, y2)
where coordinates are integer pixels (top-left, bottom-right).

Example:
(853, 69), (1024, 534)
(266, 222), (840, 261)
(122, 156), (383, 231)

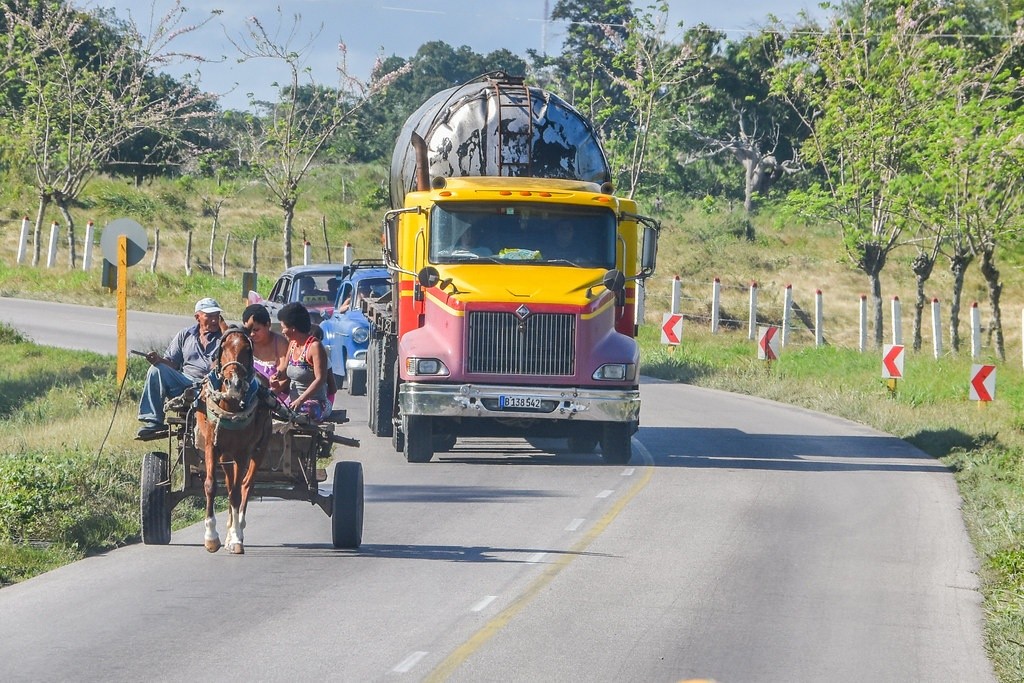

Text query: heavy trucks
(369, 67), (662, 465)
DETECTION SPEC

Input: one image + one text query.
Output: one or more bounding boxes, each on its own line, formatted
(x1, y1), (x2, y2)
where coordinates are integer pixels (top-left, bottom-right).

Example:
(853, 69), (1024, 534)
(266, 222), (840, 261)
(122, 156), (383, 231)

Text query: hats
(195, 298), (224, 315)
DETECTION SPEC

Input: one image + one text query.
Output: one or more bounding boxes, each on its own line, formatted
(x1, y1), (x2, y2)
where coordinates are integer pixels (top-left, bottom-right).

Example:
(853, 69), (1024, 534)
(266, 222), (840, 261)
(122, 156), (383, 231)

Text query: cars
(259, 258), (393, 396)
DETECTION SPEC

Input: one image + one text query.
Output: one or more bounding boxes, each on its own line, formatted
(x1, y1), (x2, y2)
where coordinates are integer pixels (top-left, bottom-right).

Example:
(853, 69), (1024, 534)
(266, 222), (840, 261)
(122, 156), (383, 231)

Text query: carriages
(139, 323), (368, 557)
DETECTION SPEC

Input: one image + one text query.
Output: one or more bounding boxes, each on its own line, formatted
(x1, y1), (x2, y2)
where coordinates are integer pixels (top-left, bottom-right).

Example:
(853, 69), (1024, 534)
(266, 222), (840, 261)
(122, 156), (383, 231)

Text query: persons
(137, 298), (229, 436)
(655, 197), (660, 211)
(277, 302), (327, 421)
(542, 219), (595, 260)
(308, 323), (337, 418)
(339, 286), (371, 313)
(242, 304), (288, 403)
(440, 229), (493, 256)
(304, 277), (340, 301)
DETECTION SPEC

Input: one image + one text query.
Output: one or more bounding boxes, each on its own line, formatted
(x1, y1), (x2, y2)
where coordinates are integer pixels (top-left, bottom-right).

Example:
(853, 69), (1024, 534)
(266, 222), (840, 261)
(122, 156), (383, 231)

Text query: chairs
(303, 277), (319, 304)
(327, 278), (341, 303)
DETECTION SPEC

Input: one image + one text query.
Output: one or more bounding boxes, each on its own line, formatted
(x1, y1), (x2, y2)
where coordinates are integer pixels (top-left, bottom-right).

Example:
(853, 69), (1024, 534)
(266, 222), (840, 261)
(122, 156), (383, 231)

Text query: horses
(192, 314), (275, 555)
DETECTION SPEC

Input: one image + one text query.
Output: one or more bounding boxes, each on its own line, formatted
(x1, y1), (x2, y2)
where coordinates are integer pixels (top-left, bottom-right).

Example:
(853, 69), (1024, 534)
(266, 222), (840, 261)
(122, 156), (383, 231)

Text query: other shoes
(138, 421), (164, 436)
